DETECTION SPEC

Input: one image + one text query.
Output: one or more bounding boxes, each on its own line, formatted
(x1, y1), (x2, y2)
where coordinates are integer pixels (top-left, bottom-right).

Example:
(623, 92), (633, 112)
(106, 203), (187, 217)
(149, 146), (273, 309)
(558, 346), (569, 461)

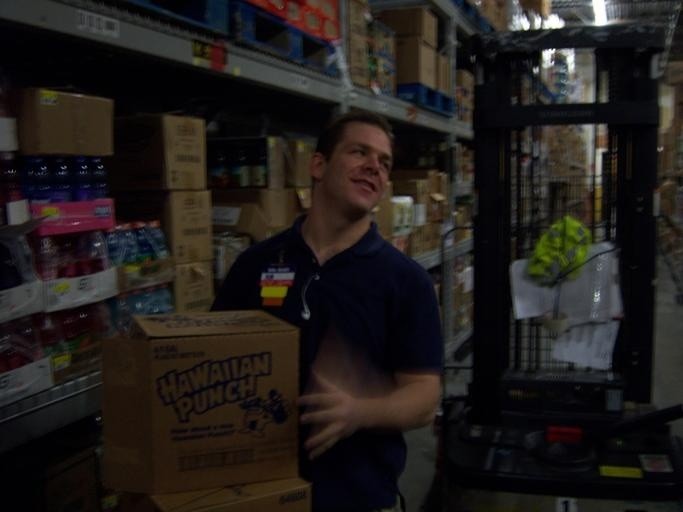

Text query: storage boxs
(97, 310), (298, 492)
(115, 476), (309, 512)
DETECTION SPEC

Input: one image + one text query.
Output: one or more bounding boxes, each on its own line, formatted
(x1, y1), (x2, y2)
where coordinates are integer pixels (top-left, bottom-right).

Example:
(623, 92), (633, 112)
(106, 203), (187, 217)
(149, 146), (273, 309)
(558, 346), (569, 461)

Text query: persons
(210, 109), (445, 512)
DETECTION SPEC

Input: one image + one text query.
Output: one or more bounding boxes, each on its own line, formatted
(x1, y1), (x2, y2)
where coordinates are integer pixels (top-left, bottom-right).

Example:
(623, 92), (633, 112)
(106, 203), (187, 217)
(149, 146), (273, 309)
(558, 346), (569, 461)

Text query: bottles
(114, 283), (174, 332)
(1, 220), (171, 291)
(0, 305), (114, 374)
(210, 141), (268, 188)
(0, 153), (112, 221)
